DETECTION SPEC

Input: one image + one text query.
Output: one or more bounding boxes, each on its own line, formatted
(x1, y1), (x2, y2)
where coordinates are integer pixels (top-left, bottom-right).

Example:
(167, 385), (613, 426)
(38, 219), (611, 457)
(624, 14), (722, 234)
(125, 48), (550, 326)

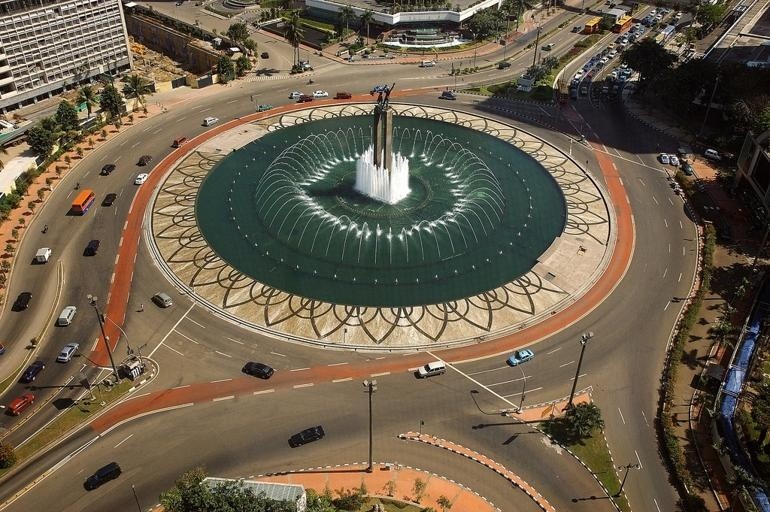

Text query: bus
(555, 79), (569, 103)
(71, 189), (96, 215)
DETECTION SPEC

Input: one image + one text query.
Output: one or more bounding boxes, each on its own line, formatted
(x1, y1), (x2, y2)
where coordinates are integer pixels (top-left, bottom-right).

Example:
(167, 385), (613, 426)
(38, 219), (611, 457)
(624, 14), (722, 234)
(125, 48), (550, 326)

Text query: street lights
(617, 462), (640, 497)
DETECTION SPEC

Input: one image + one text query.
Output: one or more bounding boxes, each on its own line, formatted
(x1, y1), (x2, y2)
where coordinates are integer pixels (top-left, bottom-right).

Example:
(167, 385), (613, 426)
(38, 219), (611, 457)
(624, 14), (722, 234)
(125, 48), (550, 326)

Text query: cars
(242, 361), (274, 380)
(101, 155), (152, 207)
(569, 7), (683, 100)
(439, 91), (457, 100)
(8, 292), (79, 416)
(509, 349), (535, 365)
(499, 62), (511, 68)
(418, 361), (446, 378)
(256, 104), (272, 111)
(659, 148), (722, 175)
(84, 462), (121, 491)
(288, 425), (325, 448)
(153, 292), (173, 307)
(83, 240), (100, 256)
(288, 90), (352, 103)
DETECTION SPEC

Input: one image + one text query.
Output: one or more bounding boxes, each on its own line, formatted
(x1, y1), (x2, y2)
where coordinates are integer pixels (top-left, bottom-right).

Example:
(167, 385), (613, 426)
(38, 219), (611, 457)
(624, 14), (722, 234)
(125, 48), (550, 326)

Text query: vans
(547, 43), (556, 49)
(422, 60), (436, 68)
(204, 117), (219, 126)
(173, 136), (187, 147)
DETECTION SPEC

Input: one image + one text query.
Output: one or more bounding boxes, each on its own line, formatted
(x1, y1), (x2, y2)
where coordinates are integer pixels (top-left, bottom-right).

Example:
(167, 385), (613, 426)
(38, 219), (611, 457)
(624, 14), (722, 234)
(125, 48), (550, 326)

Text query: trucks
(36, 247), (51, 263)
